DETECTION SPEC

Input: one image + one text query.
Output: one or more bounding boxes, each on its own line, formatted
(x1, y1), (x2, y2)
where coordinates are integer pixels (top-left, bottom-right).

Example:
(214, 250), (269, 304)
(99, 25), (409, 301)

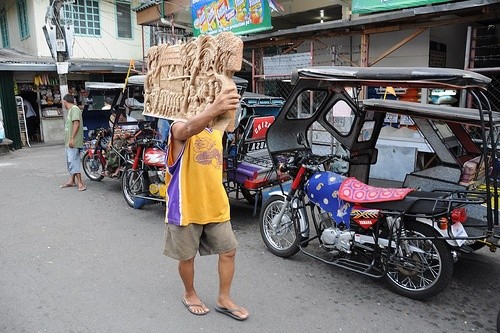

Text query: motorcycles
(81, 82), (128, 181)
(259, 63), (500, 301)
(121, 75), (296, 209)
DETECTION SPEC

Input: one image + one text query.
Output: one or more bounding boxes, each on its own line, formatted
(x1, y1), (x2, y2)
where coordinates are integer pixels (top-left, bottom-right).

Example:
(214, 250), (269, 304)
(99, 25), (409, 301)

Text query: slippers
(215, 306), (243, 321)
(60, 183), (76, 188)
(78, 184), (86, 191)
(181, 298), (209, 315)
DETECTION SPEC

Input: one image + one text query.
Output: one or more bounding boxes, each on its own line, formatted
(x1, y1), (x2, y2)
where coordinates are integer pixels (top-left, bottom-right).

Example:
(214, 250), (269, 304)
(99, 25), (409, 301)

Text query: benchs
(403, 155), (493, 190)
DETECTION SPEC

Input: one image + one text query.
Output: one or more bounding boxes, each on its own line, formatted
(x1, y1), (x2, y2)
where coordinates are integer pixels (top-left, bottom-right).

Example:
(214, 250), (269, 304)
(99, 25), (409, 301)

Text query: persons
(167, 87), (250, 321)
(60, 94), (87, 190)
(102, 98), (137, 134)
(22, 99), (38, 141)
